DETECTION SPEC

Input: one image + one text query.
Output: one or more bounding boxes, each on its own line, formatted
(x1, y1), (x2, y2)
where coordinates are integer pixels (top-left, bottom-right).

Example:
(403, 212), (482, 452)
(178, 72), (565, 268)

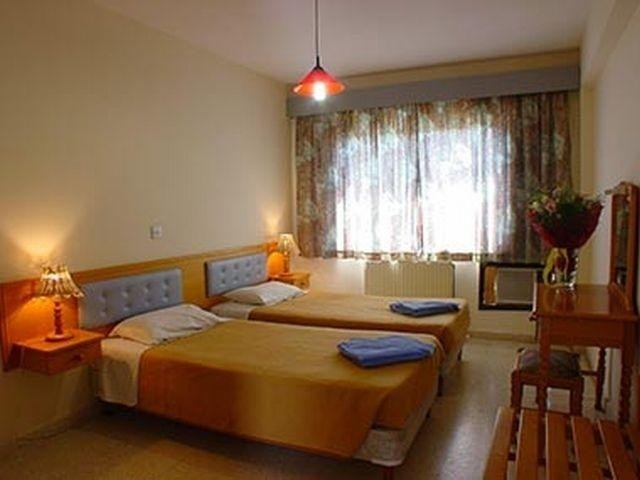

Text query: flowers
(529, 184), (602, 283)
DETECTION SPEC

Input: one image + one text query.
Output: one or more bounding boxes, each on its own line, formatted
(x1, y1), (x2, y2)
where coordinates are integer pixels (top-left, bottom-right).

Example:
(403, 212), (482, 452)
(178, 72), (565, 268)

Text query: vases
(553, 245), (579, 293)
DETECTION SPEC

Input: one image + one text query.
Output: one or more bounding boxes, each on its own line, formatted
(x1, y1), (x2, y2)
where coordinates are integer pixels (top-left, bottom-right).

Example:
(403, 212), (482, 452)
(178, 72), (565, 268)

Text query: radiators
(361, 261), (455, 300)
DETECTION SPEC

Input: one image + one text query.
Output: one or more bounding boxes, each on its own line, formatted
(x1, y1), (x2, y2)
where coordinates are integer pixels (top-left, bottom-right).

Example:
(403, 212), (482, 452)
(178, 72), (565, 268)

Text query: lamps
(274, 234), (300, 276)
(32, 263), (85, 342)
(290, 0), (344, 102)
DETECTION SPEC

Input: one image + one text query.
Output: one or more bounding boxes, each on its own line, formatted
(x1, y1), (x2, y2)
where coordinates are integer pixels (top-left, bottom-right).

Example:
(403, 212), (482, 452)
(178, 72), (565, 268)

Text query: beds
(78, 269), (440, 479)
(205, 252), (469, 394)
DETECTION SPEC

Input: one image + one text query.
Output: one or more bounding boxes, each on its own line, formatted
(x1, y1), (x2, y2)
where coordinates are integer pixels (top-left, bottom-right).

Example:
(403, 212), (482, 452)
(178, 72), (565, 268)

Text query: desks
(532, 281), (638, 428)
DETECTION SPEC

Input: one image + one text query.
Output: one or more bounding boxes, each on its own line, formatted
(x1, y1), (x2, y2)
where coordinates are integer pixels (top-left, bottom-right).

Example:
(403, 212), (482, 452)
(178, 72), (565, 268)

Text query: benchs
(483, 405), (640, 480)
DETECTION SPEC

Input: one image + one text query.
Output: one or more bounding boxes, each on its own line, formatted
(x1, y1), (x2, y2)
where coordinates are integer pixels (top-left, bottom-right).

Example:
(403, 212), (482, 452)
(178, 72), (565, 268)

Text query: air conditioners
(478, 263), (549, 311)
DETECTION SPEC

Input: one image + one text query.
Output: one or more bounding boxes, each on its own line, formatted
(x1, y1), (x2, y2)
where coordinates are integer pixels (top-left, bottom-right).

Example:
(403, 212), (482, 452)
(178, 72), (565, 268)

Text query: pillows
(106, 303), (222, 347)
(221, 280), (307, 305)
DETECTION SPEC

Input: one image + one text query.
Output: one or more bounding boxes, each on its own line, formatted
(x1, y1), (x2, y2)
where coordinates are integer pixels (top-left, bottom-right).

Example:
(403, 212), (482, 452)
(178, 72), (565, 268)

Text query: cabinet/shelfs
(19, 328), (106, 376)
(270, 272), (309, 290)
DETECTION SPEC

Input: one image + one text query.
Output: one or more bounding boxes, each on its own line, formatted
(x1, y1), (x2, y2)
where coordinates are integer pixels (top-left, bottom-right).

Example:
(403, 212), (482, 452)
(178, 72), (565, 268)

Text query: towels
(389, 297), (460, 317)
(337, 333), (436, 366)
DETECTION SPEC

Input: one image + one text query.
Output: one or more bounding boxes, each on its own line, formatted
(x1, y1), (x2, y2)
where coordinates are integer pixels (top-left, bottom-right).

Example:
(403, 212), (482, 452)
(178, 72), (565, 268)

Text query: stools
(511, 347), (583, 449)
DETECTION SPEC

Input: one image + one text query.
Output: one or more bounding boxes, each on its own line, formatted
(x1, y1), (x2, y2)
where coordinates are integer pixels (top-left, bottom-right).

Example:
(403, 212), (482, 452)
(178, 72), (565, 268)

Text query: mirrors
(607, 180), (640, 311)
